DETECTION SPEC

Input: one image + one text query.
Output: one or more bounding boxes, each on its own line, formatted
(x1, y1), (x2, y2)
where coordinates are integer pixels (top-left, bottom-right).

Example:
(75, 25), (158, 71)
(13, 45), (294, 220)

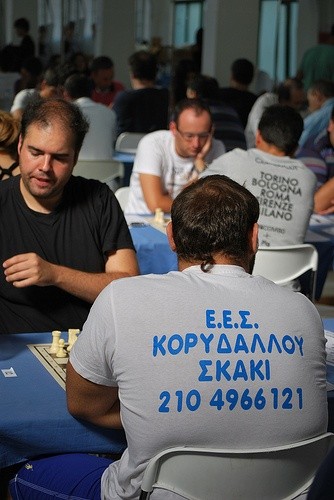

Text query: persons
(11, 174), (328, 500)
(125, 100), (226, 224)
(0, 18), (125, 113)
(113, 51), (168, 186)
(0, 98), (139, 335)
(138, 21), (334, 158)
(64, 74), (120, 192)
(298, 108), (334, 216)
(0, 109), (26, 180)
(183, 103), (317, 293)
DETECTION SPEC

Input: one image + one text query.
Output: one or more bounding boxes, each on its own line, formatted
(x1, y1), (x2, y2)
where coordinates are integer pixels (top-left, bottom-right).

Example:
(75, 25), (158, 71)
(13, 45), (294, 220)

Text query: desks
(112, 136), (137, 187)
(0, 319), (333, 461)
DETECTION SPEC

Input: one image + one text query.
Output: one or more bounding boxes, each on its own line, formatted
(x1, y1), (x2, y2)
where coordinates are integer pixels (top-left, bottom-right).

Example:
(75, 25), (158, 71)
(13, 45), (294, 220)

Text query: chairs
(139, 431), (334, 500)
(234, 244), (318, 306)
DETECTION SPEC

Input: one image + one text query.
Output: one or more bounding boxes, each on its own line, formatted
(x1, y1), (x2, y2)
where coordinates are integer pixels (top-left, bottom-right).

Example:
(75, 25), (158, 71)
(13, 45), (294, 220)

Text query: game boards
(144, 218), (172, 236)
(25, 342), (81, 391)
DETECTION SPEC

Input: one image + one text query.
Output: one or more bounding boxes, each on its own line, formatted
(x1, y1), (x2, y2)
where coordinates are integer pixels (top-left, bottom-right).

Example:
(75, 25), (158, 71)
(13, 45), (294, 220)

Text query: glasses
(176, 126), (211, 141)
